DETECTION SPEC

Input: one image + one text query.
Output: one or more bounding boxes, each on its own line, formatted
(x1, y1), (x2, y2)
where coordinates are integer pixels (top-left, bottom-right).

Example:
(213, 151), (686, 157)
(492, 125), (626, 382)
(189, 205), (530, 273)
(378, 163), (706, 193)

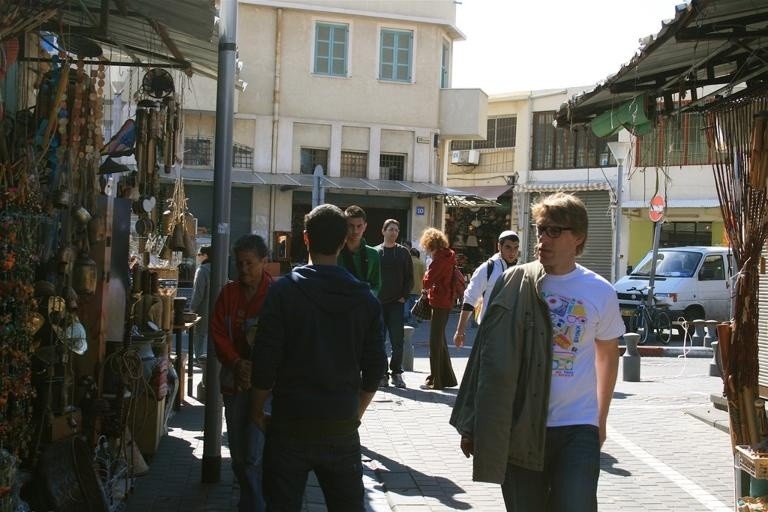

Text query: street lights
(607, 142), (628, 283)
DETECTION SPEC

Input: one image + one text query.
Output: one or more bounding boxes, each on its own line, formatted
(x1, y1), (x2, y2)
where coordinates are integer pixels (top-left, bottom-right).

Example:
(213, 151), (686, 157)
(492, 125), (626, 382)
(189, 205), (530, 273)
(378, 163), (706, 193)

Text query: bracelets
(456, 330), (466, 337)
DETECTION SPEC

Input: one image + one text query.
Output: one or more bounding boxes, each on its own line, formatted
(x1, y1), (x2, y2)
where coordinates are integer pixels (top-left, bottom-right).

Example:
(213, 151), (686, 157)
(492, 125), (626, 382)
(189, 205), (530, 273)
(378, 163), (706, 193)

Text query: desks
(172, 316), (202, 411)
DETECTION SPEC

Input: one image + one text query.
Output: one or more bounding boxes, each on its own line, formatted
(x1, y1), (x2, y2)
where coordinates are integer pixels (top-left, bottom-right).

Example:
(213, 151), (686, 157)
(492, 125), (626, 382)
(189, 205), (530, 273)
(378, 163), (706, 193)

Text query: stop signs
(648, 195), (664, 222)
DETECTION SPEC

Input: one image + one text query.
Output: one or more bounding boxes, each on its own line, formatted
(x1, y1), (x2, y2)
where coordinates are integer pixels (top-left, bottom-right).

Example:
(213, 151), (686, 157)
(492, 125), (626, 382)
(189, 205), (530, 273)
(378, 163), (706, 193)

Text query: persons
(337, 205), (458, 390)
(454, 228), (520, 350)
(209, 234), (276, 511)
(250, 203), (388, 512)
(186, 243), (211, 366)
(460, 192), (627, 512)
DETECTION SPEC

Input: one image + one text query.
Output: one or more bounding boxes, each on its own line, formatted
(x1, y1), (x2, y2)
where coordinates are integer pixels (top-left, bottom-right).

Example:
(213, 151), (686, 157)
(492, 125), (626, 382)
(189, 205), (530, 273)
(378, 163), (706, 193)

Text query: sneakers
(420, 379), (448, 390)
(185, 356), (201, 368)
(377, 372), (407, 389)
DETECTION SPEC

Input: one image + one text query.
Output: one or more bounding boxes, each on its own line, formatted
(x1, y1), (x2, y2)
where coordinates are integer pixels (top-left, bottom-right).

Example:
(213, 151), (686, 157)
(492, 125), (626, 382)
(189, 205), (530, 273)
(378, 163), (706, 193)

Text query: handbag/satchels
(410, 292), (433, 323)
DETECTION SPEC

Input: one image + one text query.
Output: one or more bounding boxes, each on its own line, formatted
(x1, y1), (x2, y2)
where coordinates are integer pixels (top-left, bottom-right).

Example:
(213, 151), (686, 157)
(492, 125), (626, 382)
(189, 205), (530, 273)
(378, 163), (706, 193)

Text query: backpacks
(451, 265), (468, 300)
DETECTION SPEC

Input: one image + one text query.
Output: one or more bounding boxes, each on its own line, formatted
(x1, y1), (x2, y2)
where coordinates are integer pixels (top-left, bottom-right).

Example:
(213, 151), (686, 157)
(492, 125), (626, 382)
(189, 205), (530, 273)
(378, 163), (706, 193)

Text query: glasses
(197, 252), (204, 257)
(531, 223), (573, 238)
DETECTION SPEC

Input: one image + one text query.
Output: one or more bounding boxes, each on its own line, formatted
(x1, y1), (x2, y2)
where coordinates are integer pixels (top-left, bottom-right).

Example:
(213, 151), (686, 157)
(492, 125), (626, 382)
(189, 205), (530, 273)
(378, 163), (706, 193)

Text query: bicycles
(626, 287), (672, 345)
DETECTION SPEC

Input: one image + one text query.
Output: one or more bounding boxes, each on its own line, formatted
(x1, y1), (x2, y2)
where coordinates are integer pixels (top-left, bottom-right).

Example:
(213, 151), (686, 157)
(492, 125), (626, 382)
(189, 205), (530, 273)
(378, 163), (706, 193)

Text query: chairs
(670, 261), (715, 280)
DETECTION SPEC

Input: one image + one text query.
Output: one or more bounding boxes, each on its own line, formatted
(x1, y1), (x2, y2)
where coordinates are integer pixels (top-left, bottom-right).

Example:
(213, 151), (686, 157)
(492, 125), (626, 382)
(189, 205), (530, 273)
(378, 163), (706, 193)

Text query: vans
(613, 245), (737, 340)
